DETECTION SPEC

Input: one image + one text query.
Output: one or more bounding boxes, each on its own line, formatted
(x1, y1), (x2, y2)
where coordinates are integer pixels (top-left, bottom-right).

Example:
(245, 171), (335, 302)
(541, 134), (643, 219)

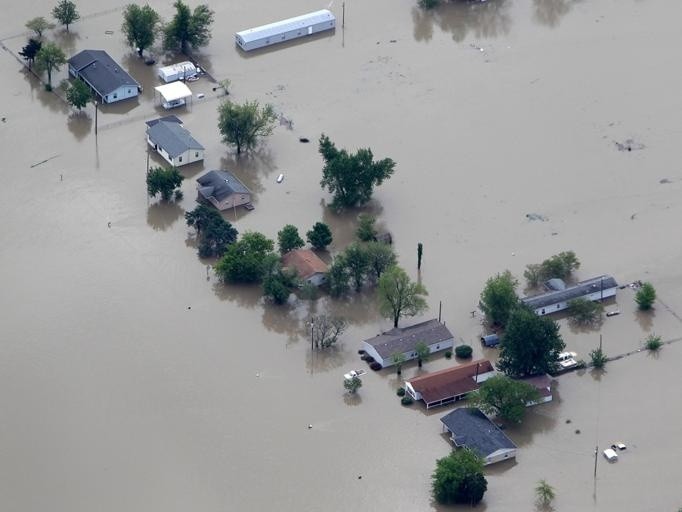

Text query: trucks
(554, 351), (577, 361)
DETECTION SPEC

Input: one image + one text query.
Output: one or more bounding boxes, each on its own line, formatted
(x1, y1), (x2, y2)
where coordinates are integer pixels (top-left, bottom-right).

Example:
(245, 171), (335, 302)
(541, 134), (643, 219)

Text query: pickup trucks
(343, 368), (369, 379)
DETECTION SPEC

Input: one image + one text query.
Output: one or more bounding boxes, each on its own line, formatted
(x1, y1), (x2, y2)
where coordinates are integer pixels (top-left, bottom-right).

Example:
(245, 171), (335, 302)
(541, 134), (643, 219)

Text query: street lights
(310, 316), (315, 350)
(592, 445), (600, 479)
(94, 95), (100, 135)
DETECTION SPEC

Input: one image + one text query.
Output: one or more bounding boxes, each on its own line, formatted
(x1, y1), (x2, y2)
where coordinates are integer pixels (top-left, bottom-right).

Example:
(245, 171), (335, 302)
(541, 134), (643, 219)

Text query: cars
(606, 310), (620, 316)
